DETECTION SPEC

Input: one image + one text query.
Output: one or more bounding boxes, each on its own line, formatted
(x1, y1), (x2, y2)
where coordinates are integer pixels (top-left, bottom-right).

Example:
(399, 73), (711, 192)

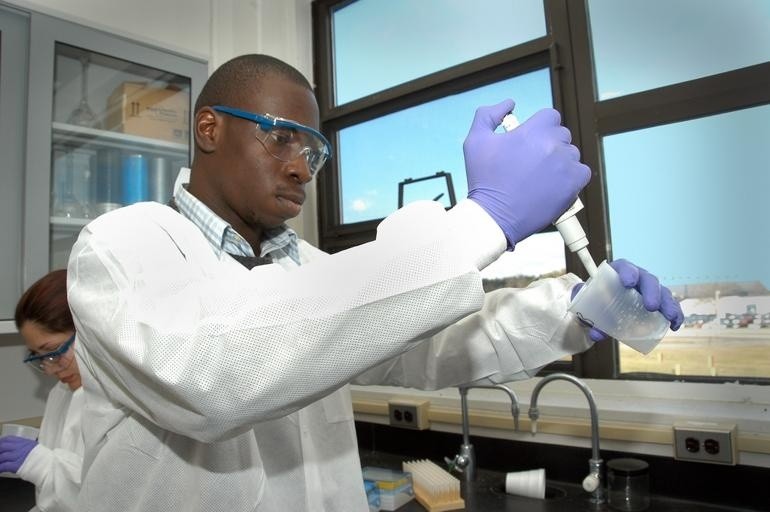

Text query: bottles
(95, 147), (172, 214)
(66, 53), (104, 129)
(605, 458), (650, 510)
(54, 142), (85, 217)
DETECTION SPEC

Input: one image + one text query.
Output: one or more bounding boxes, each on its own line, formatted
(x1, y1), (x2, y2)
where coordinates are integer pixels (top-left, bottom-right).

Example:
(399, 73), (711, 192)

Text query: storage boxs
(104, 80), (190, 146)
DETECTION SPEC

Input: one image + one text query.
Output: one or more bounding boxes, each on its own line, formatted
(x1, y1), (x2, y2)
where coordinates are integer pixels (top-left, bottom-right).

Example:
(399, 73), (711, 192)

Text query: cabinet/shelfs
(1, 0), (211, 334)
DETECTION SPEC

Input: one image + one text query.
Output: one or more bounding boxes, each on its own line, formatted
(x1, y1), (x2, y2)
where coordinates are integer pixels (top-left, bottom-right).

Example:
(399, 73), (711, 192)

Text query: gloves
(572, 260), (684, 343)
(0, 434), (40, 472)
(464, 100), (591, 253)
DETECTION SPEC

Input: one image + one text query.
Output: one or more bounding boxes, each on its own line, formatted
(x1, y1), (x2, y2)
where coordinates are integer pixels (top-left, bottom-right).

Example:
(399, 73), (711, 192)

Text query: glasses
(23, 335), (77, 378)
(210, 104), (332, 175)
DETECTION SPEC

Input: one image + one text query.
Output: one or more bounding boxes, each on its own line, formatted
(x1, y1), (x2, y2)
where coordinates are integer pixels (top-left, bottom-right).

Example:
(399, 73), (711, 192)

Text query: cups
(505, 468), (547, 498)
(1, 424), (39, 481)
(565, 259), (672, 355)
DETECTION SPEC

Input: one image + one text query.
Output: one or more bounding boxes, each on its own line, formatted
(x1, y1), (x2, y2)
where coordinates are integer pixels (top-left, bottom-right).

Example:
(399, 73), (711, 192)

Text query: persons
(0, 268), (83, 512)
(67, 53), (685, 512)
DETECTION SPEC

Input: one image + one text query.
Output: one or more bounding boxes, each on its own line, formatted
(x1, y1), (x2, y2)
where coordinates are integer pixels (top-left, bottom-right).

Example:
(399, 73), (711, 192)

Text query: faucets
(528, 374), (600, 461)
(460, 382), (520, 445)
